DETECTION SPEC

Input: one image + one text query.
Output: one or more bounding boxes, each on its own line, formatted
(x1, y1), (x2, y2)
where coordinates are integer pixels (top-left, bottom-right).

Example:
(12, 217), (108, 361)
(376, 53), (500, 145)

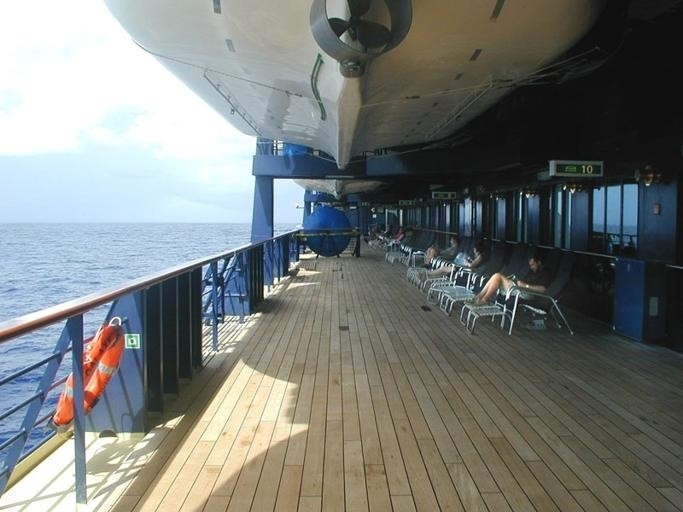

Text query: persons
(415, 245), (483, 280)
(467, 255), (550, 306)
(421, 237), (458, 268)
(375, 225), (405, 245)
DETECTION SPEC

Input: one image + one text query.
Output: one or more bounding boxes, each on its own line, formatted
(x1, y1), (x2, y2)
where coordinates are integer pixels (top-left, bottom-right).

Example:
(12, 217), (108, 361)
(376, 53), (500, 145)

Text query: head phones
(538, 259), (543, 266)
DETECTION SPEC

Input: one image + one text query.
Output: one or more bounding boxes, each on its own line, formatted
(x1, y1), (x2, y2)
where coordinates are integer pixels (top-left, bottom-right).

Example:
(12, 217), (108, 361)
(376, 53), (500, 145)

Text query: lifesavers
(53, 325), (125, 426)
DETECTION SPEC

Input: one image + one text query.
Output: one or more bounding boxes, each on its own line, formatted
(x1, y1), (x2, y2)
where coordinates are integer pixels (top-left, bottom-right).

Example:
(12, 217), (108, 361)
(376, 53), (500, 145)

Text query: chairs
(366, 224), (576, 337)
